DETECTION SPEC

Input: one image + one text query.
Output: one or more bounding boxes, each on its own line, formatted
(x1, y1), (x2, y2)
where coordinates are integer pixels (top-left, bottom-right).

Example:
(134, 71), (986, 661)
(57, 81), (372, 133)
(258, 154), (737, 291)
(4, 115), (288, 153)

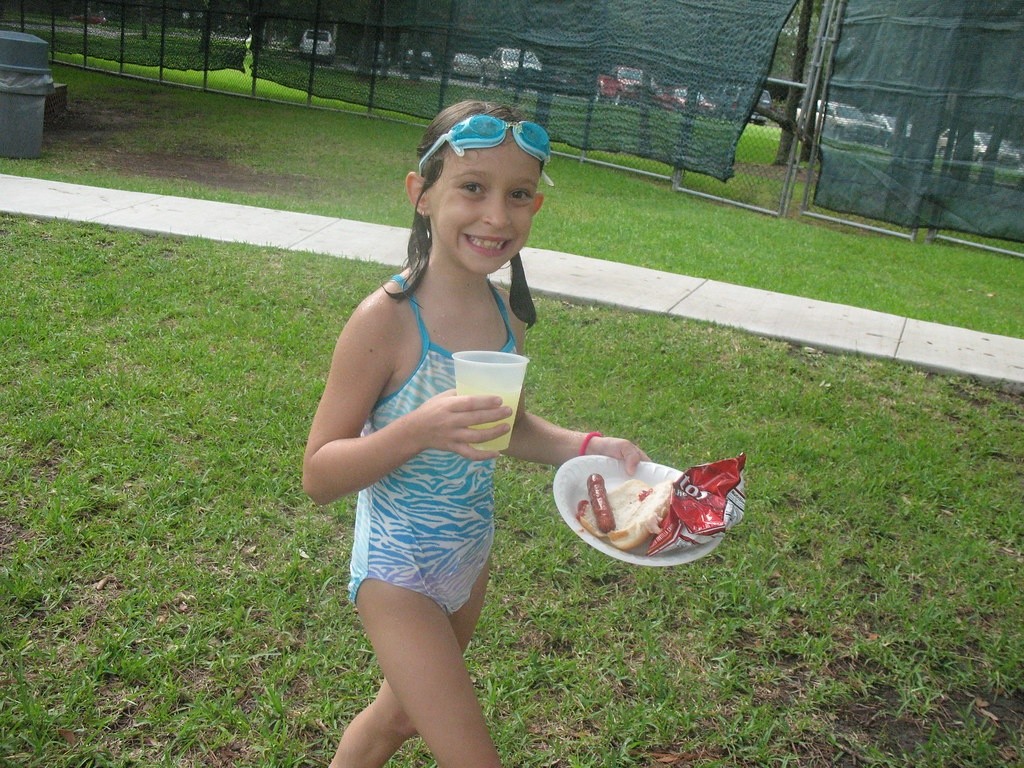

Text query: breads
(579, 479), (671, 549)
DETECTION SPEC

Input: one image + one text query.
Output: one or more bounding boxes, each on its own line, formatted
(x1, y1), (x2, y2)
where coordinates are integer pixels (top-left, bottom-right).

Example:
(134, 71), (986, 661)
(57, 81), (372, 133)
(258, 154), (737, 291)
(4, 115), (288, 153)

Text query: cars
(351, 42), (392, 70)
(482, 49), (541, 88)
(795, 99), (1020, 167)
(69, 12), (106, 26)
(544, 63), (772, 125)
(453, 53), (484, 82)
(397, 49), (436, 79)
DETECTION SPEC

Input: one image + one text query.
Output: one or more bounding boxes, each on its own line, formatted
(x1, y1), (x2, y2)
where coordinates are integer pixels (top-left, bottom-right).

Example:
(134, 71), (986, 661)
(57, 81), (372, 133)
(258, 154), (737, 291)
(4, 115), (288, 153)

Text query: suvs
(300, 30), (338, 65)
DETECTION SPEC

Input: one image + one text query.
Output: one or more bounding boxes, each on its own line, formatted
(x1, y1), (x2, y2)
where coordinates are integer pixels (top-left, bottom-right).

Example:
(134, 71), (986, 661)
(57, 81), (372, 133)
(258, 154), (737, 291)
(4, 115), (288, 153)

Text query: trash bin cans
(0, 30), (56, 158)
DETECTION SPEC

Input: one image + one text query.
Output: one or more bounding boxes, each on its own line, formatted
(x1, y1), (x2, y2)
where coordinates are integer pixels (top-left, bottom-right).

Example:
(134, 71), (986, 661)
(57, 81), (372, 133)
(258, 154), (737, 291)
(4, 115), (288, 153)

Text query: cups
(454, 352), (529, 451)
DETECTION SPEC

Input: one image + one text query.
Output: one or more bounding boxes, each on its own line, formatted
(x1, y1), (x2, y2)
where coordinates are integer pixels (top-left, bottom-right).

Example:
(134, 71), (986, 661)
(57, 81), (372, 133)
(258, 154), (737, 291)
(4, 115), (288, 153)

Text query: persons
(301, 98), (653, 768)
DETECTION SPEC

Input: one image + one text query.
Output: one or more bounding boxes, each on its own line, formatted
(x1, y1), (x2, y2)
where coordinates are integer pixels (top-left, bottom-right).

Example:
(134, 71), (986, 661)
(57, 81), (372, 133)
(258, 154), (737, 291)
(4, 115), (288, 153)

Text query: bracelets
(579, 432), (602, 457)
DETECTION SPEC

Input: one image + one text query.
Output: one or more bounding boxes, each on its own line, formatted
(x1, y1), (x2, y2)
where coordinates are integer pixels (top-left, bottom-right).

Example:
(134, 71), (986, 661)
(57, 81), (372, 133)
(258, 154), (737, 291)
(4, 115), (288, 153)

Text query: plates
(553, 455), (724, 567)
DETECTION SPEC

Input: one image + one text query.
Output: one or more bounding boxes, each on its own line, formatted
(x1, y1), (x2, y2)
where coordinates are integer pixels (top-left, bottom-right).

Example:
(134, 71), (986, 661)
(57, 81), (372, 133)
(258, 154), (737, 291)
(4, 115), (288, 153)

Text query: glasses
(419, 113), (552, 176)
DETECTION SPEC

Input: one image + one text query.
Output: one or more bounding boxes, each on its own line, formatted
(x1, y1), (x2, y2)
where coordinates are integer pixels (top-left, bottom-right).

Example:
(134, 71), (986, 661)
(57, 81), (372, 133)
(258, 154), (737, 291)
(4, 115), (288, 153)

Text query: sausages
(587, 473), (616, 533)
